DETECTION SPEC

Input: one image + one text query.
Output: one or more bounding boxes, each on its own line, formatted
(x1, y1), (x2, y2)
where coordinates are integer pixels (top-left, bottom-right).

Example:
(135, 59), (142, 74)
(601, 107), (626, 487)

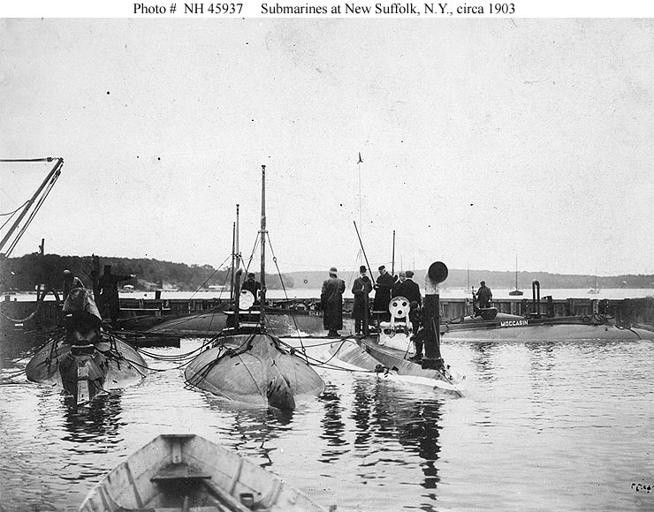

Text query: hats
(328, 266), (412, 279)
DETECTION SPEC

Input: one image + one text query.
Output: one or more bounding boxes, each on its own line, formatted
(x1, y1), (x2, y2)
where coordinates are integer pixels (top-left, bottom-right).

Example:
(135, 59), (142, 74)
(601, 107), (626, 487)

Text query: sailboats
(179, 165), (330, 409)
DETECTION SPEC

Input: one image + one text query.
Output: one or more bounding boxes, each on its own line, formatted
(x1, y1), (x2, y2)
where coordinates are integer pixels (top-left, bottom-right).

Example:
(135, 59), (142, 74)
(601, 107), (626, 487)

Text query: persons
(392, 272), (406, 294)
(320, 267), (346, 337)
(241, 273), (268, 303)
(62, 270), (85, 303)
(373, 265), (394, 312)
(474, 281), (492, 308)
(99, 264), (137, 320)
(408, 299), (426, 360)
(351, 265), (373, 336)
(395, 270), (423, 309)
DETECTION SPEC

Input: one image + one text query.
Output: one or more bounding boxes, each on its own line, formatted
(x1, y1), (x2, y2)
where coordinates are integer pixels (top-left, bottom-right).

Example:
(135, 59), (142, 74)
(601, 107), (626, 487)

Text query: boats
(444, 280), (651, 348)
(74, 431), (331, 510)
(325, 220), (464, 404)
(19, 327), (145, 415)
